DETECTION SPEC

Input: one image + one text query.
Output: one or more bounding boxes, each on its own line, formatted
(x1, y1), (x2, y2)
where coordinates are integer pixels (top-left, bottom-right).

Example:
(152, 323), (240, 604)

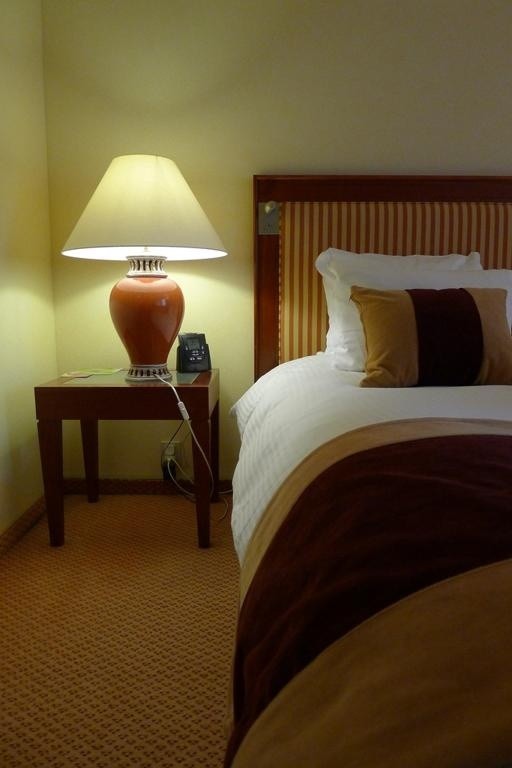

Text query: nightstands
(34, 372), (219, 547)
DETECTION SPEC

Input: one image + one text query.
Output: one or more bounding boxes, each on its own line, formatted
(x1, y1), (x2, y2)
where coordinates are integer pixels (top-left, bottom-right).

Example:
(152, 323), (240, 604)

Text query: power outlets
(160, 440), (182, 468)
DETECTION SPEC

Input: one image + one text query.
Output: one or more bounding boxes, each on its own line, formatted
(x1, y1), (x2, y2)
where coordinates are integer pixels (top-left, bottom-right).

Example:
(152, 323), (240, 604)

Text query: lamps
(60, 153), (227, 381)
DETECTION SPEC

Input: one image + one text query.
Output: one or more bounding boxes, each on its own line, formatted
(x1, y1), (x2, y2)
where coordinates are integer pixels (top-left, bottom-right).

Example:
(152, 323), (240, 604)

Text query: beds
(227, 176), (512, 768)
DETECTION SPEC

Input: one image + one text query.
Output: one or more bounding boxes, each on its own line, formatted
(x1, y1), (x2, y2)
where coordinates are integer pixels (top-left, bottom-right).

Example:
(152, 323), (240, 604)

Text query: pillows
(350, 285), (512, 387)
(386, 270), (512, 332)
(315, 246), (483, 371)
